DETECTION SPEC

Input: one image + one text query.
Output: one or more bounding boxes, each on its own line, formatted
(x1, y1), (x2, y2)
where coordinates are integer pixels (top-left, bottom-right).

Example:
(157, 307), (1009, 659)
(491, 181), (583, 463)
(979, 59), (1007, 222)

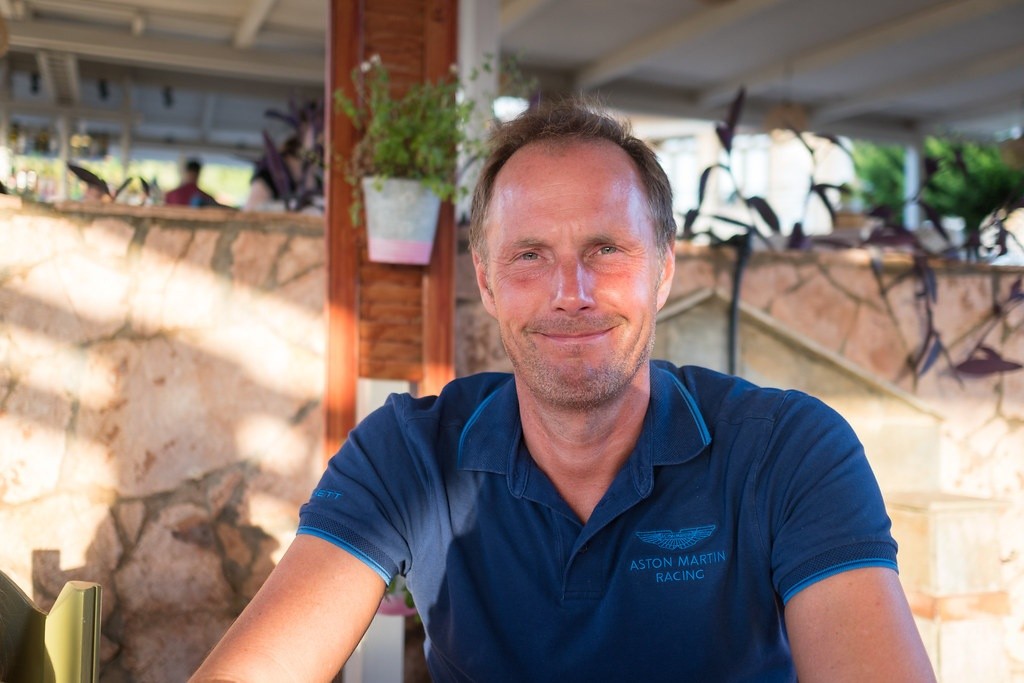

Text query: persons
(184, 98), (941, 683)
(83, 183), (107, 204)
(787, 221), (812, 251)
(163, 157), (217, 208)
(244, 133), (325, 218)
(869, 204), (918, 246)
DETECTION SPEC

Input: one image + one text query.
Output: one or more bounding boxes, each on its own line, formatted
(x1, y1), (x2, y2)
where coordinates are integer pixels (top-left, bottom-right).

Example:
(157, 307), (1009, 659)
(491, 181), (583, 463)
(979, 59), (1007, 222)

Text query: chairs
(1, 567), (104, 682)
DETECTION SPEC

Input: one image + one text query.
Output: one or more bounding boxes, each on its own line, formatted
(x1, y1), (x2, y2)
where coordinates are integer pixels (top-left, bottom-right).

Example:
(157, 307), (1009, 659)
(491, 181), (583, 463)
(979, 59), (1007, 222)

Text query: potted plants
(331, 49), (502, 266)
